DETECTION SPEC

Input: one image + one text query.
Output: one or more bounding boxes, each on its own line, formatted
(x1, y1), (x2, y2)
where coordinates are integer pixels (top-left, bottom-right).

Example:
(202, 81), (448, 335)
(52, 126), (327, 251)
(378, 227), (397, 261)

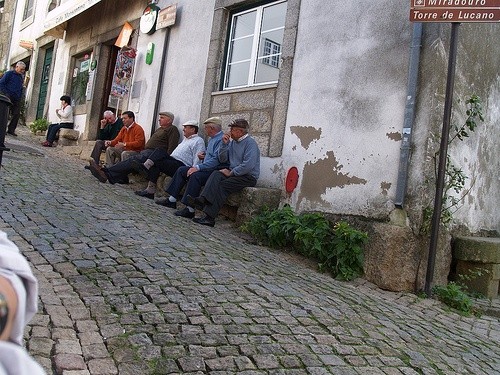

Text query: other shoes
(84, 164), (90, 168)
(8, 131), (17, 136)
(41, 141), (51, 146)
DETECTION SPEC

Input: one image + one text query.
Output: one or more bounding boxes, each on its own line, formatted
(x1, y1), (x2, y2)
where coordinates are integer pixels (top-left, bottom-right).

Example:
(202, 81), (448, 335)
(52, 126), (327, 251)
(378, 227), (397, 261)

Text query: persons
(0, 226), (49, 375)
(42, 96), (74, 147)
(84, 110), (261, 227)
(0, 61), (29, 151)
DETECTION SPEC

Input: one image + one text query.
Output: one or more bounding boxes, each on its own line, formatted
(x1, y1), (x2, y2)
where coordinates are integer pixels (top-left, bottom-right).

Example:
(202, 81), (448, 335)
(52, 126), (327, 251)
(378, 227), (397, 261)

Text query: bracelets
(0, 289), (8, 335)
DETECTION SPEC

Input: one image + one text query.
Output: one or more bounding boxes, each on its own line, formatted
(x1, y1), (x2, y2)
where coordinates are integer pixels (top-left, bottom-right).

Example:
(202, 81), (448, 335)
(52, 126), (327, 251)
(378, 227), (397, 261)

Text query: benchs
(157, 172), (172, 189)
(223, 186), (281, 232)
(59, 127), (78, 145)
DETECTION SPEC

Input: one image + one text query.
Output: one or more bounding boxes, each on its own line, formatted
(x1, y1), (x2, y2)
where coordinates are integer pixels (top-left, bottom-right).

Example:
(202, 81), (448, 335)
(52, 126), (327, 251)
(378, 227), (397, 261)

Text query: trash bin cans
(0, 95), (14, 167)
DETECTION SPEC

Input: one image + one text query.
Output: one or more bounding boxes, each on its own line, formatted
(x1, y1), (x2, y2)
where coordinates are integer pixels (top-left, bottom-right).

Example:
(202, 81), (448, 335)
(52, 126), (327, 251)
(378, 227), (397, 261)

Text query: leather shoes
(192, 215), (216, 227)
(88, 164), (109, 183)
(134, 188), (154, 199)
(155, 196), (176, 208)
(174, 207), (195, 218)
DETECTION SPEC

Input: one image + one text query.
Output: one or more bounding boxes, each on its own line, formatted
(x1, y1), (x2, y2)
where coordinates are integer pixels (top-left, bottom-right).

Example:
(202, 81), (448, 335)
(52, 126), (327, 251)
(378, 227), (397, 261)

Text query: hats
(228, 118), (249, 129)
(202, 116), (222, 126)
(158, 111), (175, 120)
(182, 120), (199, 126)
